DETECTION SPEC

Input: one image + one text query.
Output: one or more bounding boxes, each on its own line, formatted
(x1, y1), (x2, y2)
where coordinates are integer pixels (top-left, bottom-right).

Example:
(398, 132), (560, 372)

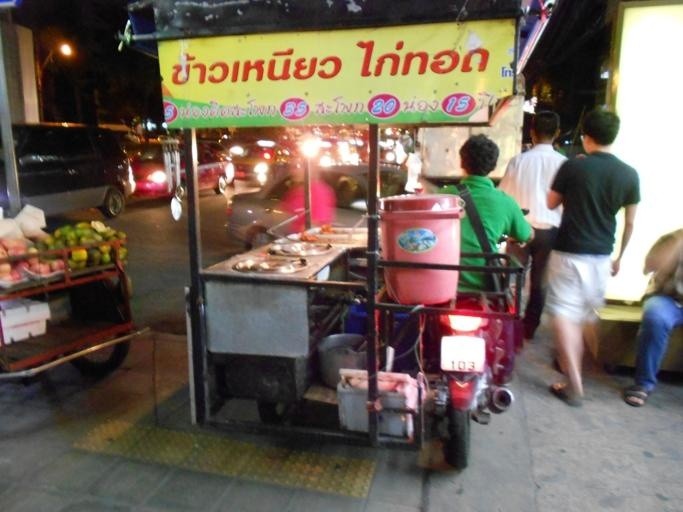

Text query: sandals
(625, 385), (649, 406)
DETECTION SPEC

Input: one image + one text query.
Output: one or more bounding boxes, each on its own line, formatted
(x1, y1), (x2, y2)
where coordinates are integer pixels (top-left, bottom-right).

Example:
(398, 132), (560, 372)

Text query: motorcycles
(413, 206), (528, 467)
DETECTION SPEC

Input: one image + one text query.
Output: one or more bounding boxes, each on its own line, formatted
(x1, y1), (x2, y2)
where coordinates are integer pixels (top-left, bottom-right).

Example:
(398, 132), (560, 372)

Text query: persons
(437, 132), (535, 292)
(620, 223), (682, 408)
(547, 107), (641, 406)
(271, 154), (336, 233)
(498, 111), (570, 341)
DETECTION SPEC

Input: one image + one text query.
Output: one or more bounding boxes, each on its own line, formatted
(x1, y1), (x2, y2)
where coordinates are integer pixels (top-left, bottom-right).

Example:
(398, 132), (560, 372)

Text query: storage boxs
(335, 367), (420, 441)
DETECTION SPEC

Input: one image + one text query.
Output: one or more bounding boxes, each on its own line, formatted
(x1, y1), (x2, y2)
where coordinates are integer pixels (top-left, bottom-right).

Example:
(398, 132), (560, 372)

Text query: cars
(220, 125), (411, 187)
(521, 127), (585, 159)
(226, 165), (405, 257)
(204, 141), (231, 160)
(130, 140), (234, 201)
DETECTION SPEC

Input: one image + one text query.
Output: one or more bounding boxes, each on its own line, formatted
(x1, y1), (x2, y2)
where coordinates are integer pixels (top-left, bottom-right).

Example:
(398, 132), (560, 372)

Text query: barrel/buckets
(316, 302), (415, 390)
(376, 194), (465, 306)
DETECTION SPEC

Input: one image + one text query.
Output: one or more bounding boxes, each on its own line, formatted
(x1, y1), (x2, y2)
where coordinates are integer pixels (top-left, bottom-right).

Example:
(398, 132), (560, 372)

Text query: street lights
(34, 42), (72, 85)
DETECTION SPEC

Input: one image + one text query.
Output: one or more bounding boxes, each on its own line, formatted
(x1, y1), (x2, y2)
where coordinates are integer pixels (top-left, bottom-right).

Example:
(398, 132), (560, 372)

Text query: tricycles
(0, 237), (150, 381)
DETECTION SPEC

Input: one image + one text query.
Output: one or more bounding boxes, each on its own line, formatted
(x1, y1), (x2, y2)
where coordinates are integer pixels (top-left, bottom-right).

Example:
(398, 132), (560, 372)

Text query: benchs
(584, 303), (682, 375)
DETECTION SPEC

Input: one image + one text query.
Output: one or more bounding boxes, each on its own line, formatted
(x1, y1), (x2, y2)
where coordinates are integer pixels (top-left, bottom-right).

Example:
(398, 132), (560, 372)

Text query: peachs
(0, 239), (65, 281)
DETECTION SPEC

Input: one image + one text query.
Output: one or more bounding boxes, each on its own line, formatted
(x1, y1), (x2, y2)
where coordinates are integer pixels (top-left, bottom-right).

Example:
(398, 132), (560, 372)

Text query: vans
(0, 122), (135, 220)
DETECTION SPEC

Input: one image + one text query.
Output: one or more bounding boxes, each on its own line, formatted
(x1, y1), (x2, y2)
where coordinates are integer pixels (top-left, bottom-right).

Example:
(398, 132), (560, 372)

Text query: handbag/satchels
(486, 260), (516, 306)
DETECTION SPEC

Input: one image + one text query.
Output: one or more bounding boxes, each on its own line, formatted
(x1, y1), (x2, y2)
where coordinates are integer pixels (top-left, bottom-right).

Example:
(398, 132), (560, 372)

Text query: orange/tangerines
(26, 221), (128, 270)
